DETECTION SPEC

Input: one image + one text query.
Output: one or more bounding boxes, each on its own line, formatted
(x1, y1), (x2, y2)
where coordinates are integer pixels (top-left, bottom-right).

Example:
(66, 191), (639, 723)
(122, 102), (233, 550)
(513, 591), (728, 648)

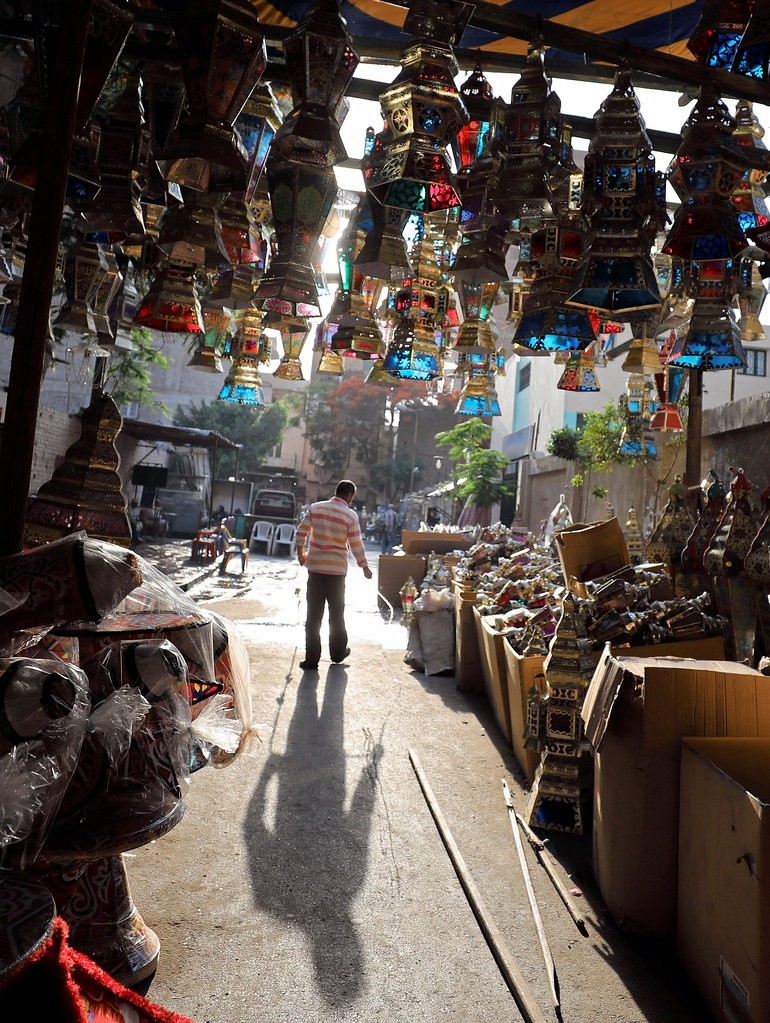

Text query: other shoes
(300, 661), (318, 670)
(334, 647), (350, 663)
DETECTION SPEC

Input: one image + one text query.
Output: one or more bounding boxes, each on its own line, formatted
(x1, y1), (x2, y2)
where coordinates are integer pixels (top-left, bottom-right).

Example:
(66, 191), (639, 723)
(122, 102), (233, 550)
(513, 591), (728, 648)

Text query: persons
(296, 480), (372, 670)
(212, 504), (226, 522)
(364, 504), (399, 556)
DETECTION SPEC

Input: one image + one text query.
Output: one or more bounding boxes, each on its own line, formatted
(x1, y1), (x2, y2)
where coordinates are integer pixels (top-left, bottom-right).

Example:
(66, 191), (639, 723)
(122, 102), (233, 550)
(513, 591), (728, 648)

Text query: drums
(0, 526), (255, 1023)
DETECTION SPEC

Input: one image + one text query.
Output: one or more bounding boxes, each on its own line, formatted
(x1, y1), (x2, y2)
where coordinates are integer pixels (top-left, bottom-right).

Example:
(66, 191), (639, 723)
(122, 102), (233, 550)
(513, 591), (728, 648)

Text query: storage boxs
(378, 517), (770, 1023)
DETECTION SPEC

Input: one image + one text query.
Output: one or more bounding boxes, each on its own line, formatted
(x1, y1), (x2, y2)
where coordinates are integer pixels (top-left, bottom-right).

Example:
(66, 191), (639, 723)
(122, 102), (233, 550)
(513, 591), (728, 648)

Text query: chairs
(248, 521), (274, 555)
(272, 524), (297, 556)
(191, 517), (249, 574)
